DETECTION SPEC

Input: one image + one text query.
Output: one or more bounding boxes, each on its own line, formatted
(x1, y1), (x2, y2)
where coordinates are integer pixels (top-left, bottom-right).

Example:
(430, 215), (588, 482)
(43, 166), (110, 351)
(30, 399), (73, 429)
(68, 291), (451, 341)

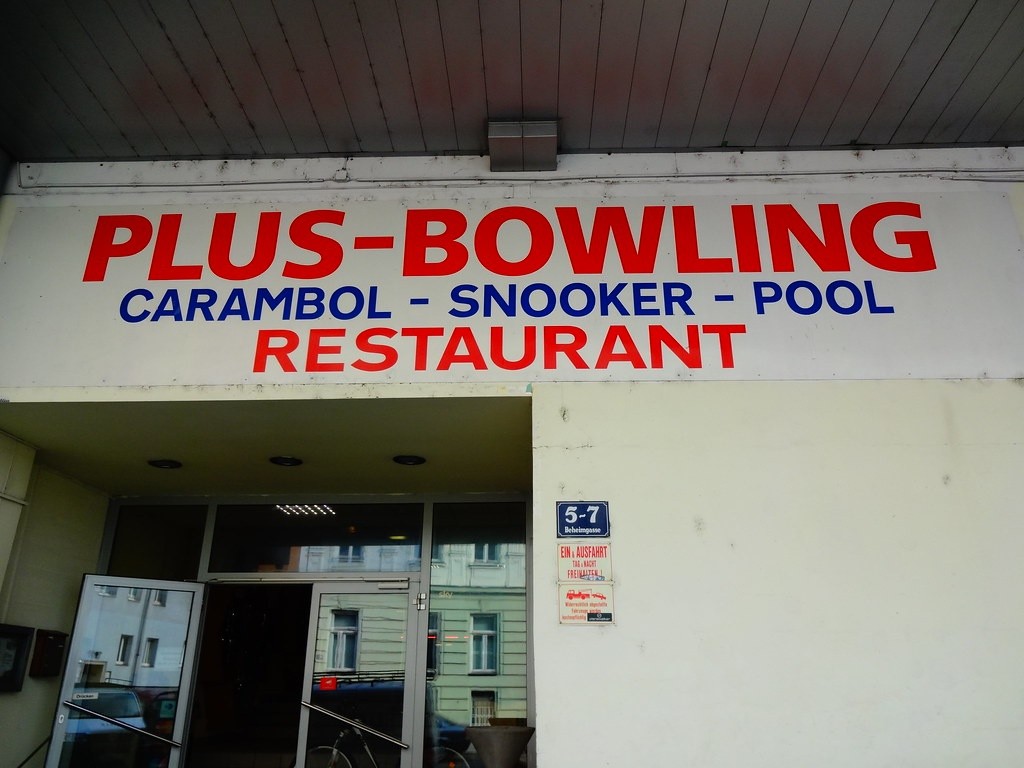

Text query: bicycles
(288, 720), (470, 768)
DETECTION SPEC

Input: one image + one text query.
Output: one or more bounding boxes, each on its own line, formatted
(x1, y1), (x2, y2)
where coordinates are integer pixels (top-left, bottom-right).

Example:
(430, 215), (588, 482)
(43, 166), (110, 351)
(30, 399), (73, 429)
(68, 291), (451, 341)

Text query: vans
(310, 678), (439, 766)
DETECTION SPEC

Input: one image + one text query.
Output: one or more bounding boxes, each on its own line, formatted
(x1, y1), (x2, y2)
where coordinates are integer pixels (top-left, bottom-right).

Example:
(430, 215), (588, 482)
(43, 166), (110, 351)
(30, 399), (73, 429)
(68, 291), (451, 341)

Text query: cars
(64, 685), (147, 767)
(438, 716), (472, 756)
(132, 688), (179, 767)
